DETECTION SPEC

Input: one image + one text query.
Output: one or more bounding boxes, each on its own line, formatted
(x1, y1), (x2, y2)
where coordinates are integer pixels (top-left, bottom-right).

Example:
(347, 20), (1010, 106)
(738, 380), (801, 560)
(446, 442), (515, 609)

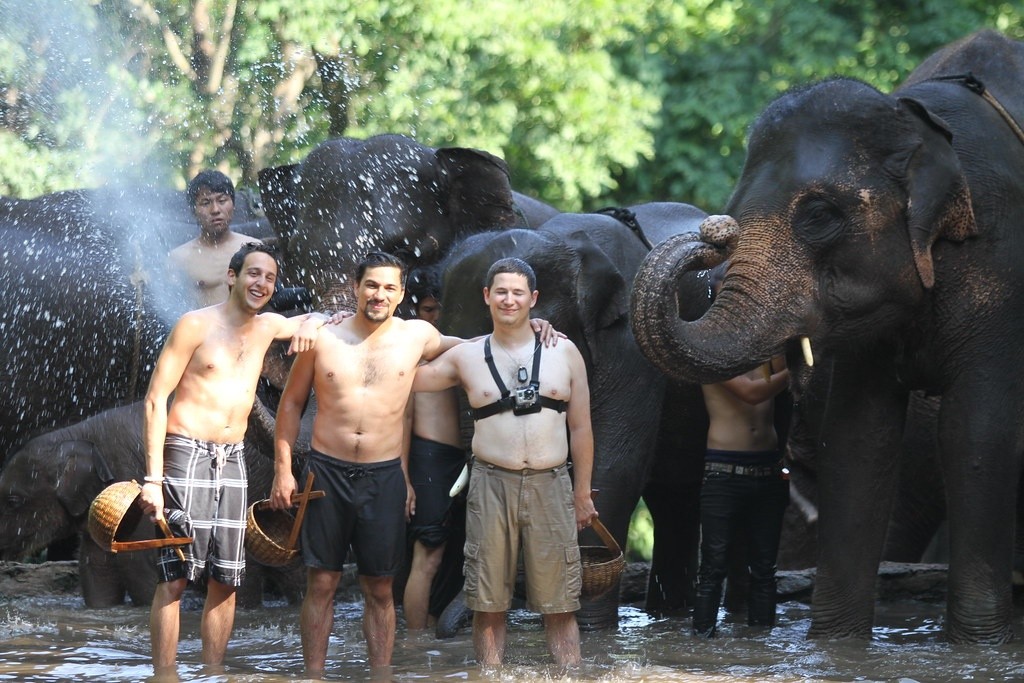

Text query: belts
(704, 462), (782, 477)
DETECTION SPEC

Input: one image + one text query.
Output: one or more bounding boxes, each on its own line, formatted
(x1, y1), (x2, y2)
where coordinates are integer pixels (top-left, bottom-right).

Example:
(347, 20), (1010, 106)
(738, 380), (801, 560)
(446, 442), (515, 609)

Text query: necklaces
(493, 331), (542, 383)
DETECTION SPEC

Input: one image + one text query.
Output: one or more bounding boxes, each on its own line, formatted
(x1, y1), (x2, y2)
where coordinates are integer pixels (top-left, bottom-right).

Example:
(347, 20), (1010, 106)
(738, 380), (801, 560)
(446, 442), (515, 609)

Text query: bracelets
(144, 477), (165, 481)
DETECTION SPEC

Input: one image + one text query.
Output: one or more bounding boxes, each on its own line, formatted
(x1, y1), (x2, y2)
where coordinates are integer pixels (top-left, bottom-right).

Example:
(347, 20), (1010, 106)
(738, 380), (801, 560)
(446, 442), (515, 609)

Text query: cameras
(516, 387), (536, 406)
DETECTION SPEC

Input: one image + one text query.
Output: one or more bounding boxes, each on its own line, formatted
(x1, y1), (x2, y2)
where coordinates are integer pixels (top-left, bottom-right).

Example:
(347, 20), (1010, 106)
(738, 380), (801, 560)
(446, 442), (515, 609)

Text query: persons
(328, 257), (599, 666)
(270, 251), (568, 670)
(139, 241), (332, 668)
(401, 264), (466, 629)
(693, 263), (792, 638)
(128, 171), (265, 313)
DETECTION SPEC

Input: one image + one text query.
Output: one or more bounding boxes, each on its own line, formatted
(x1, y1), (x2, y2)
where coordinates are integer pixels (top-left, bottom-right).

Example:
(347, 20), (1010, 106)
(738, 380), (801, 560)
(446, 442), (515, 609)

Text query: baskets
(574, 488), (624, 600)
(244, 472), (325, 567)
(87, 480), (194, 562)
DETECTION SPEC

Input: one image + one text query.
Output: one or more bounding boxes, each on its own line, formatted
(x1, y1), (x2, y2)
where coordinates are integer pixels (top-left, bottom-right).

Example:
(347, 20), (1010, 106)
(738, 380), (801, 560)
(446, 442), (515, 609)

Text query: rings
(582, 527), (585, 529)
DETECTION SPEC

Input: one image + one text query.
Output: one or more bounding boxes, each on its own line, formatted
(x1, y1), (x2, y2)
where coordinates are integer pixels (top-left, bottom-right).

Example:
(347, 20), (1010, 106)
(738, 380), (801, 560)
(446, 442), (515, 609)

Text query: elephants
(0, 131), (798, 641)
(626, 25), (1023, 649)
